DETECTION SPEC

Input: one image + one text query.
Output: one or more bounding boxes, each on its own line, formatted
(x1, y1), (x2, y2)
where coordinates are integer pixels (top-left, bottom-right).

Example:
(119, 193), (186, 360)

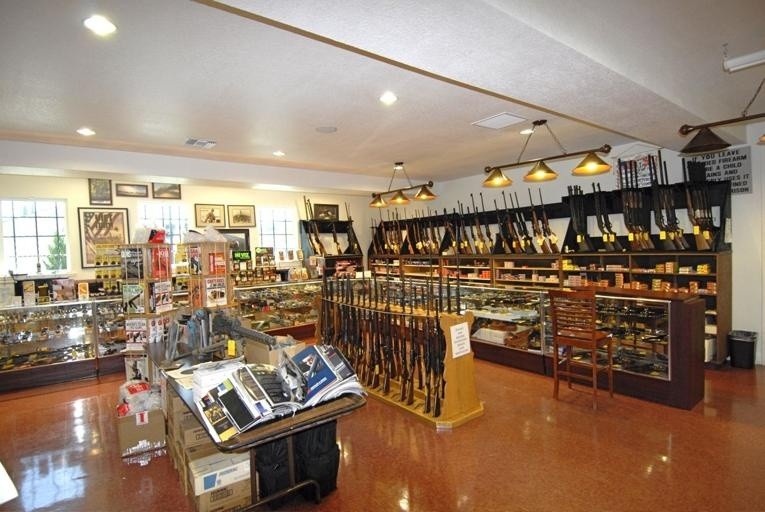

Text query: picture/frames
(228, 205), (257, 227)
(77, 208), (130, 269)
(116, 183), (148, 197)
(195, 203), (226, 228)
(314, 204), (339, 221)
(152, 182), (181, 199)
(215, 228), (249, 251)
(88, 178), (112, 206)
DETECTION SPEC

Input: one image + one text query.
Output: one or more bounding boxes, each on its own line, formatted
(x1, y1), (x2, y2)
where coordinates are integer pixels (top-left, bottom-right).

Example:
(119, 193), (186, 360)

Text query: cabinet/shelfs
(299, 220), (363, 275)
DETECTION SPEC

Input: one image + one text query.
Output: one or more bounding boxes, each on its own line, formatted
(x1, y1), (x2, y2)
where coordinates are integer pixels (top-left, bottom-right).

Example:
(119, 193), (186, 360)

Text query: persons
(202, 208), (216, 223)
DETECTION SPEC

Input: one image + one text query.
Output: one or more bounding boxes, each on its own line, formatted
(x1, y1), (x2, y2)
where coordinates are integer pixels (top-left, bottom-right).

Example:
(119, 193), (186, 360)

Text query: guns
(646, 149), (689, 251)
(677, 156), (720, 251)
(369, 188), (561, 254)
(303, 194), (318, 254)
(567, 184), (598, 255)
(211, 311), (276, 357)
(318, 273), (460, 418)
(344, 202), (360, 254)
(590, 182), (624, 253)
(329, 211), (343, 255)
(616, 157), (654, 252)
(191, 258), (202, 274)
(123, 290), (144, 312)
(307, 198), (327, 256)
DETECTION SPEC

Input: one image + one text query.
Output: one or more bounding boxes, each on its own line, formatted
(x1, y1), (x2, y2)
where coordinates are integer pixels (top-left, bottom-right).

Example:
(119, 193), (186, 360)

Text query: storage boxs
(113, 313), (306, 512)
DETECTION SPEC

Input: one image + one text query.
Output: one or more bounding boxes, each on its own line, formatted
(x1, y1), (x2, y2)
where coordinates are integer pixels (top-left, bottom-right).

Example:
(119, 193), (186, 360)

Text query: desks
(141, 339), (366, 511)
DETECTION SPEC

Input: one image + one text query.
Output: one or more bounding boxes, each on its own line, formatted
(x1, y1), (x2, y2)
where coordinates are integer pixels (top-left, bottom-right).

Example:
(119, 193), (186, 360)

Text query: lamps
(723, 49), (764, 72)
(481, 120), (612, 188)
(369, 162), (440, 208)
(679, 78), (765, 154)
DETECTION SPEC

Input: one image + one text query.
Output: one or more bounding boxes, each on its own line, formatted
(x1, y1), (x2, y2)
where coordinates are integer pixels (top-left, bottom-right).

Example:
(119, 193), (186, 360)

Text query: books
(117, 246), (229, 387)
(193, 343), (370, 444)
